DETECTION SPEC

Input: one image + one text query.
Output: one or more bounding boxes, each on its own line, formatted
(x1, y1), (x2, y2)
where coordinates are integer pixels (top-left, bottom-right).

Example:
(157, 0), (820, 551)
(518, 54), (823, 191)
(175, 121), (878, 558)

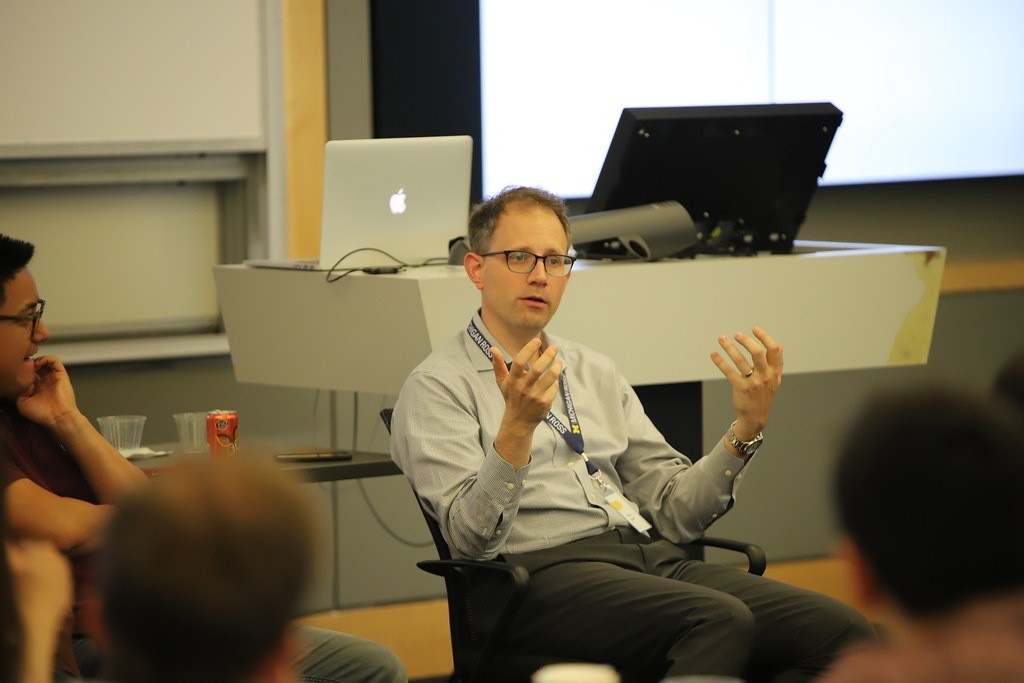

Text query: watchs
(728, 420), (764, 455)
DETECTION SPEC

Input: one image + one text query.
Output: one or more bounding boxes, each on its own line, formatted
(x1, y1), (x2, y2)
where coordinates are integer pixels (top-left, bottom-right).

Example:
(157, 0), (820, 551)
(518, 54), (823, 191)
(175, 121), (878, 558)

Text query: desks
(213, 237), (946, 398)
(131, 441), (404, 484)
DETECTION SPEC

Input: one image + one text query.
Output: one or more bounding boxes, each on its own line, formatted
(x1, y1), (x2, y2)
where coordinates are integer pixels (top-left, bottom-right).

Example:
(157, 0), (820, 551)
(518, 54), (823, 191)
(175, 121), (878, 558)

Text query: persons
(98, 452), (322, 683)
(391, 185), (878, 683)
(831, 378), (1023, 681)
(1, 231), (406, 683)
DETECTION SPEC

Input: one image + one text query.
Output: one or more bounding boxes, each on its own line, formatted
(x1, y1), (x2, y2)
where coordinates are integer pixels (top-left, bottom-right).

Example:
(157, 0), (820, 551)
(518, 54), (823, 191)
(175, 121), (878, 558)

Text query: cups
(173, 412), (208, 450)
(97, 415), (146, 451)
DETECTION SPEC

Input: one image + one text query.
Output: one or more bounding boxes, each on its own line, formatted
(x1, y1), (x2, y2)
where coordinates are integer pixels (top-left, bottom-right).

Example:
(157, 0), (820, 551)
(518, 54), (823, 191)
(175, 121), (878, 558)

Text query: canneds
(205, 409), (238, 456)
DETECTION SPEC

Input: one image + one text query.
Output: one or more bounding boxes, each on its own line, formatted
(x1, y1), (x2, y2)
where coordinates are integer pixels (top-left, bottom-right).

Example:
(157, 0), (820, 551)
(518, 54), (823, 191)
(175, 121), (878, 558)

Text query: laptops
(241, 136), (475, 272)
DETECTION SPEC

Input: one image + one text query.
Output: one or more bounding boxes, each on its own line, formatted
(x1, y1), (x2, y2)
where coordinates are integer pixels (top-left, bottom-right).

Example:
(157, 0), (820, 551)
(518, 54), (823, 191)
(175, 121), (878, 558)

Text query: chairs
(375, 407), (767, 683)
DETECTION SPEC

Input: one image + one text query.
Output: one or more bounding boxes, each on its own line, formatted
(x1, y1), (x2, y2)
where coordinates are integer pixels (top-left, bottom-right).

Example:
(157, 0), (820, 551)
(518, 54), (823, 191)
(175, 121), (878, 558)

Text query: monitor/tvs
(581, 103), (842, 257)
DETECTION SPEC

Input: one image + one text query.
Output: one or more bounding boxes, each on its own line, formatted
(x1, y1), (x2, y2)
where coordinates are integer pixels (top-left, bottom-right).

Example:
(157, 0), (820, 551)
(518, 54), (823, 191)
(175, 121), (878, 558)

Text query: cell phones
(272, 450), (353, 464)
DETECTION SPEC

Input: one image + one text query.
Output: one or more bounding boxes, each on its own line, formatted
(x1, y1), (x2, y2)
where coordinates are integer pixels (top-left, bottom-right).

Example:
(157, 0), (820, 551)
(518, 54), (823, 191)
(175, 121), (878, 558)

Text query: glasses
(480, 249), (577, 278)
(0, 300), (45, 332)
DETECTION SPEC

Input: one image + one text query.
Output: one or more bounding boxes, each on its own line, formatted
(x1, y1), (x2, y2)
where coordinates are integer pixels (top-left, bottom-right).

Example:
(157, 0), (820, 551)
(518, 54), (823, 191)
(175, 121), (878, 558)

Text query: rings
(744, 370), (753, 377)
